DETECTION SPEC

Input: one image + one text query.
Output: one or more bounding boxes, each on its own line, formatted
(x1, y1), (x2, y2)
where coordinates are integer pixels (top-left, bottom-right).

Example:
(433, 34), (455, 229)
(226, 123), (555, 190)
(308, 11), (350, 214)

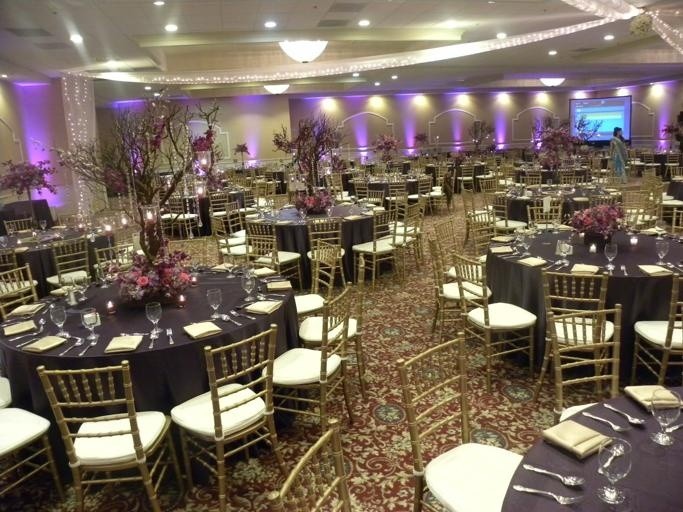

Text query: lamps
(263, 84), (289, 95)
(277, 41), (328, 64)
(539, 78), (565, 87)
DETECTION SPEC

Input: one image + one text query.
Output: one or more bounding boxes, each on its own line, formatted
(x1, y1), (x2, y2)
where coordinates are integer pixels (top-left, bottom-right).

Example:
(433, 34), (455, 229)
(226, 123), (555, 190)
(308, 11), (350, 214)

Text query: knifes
(496, 253), (520, 260)
(119, 332), (148, 336)
(1, 315), (44, 347)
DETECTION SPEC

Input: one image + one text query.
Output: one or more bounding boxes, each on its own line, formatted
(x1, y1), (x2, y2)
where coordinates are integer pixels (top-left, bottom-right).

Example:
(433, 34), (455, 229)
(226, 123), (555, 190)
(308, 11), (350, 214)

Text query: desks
(11, 265), (296, 432)
(499, 389), (683, 511)
(489, 221), (683, 360)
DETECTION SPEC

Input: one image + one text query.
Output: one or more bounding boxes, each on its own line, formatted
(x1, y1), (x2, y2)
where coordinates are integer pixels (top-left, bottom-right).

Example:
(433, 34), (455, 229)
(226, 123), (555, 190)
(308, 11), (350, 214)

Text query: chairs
(0, 375), (61, 509)
(174, 322), (293, 509)
(486, 149), (683, 227)
(120, 151), (487, 286)
(447, 247), (537, 382)
(295, 237), (338, 316)
(0, 263), (39, 318)
(259, 281), (359, 435)
(525, 203), (550, 233)
(427, 236), (492, 332)
(432, 220), (487, 280)
(543, 302), (623, 420)
(633, 262), (683, 386)
(264, 418), (353, 512)
(394, 331), (522, 511)
(467, 209), (497, 258)
(168, 236), (212, 268)
(239, 220), (278, 274)
(1, 216), (119, 285)
(35, 357), (175, 511)
(90, 243), (134, 283)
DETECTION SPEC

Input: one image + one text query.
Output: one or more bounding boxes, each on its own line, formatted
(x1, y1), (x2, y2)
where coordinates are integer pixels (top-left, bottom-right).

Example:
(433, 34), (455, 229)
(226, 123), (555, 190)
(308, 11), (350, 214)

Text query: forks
(509, 484), (588, 507)
(165, 328), (174, 345)
(582, 412), (632, 434)
(230, 309), (256, 320)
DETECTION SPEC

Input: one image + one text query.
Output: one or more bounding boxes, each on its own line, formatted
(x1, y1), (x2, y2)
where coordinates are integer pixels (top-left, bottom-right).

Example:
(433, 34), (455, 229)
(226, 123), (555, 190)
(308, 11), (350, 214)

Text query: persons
(609, 127), (629, 183)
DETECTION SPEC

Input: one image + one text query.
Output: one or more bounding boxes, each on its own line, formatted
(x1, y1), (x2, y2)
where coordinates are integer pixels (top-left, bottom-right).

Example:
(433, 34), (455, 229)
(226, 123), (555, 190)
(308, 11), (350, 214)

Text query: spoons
(221, 315), (242, 326)
(602, 402), (648, 426)
(520, 460), (588, 491)
(41, 303), (55, 314)
(254, 276), (286, 301)
(77, 337), (98, 357)
(148, 332), (158, 348)
(58, 337), (83, 356)
(234, 302), (251, 308)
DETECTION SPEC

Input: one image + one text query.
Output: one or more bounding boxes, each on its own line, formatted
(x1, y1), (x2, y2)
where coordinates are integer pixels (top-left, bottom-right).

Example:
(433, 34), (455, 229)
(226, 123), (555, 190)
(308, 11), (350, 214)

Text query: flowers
(103, 87), (215, 202)
(369, 133), (400, 153)
(271, 116), (345, 153)
(531, 128), (578, 152)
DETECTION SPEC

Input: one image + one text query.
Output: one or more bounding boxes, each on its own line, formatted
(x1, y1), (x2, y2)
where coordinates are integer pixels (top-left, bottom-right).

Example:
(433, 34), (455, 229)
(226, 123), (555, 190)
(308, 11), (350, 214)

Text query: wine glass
(1, 213), (116, 247)
(206, 288), (222, 319)
(511, 221), (683, 275)
(650, 387), (683, 446)
(258, 195), (366, 222)
(592, 436), (632, 503)
(79, 307), (98, 339)
(361, 169), (415, 183)
(144, 302), (163, 334)
(222, 253), (257, 301)
(48, 264), (108, 338)
(507, 179), (601, 198)
